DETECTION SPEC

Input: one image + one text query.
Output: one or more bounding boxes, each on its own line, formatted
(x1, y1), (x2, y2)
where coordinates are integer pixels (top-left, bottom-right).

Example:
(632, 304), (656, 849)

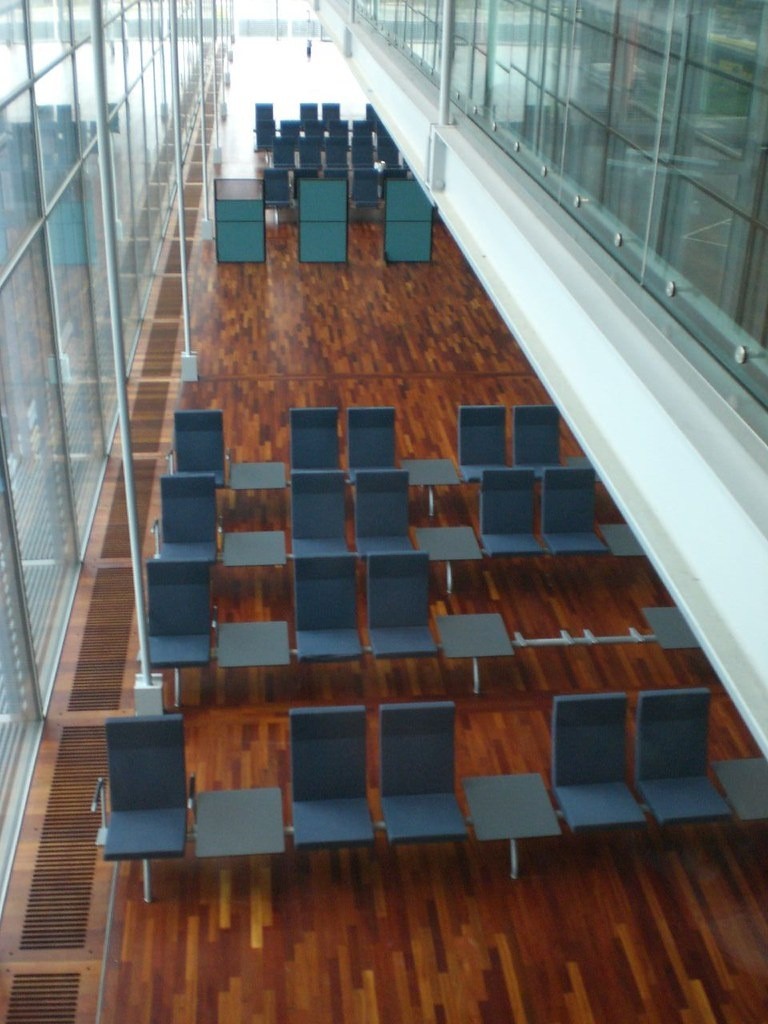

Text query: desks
(566, 454), (602, 485)
(436, 614), (514, 694)
(229, 462), (286, 489)
(459, 774), (563, 878)
(400, 459), (460, 517)
(221, 533), (287, 567)
(217, 621), (290, 665)
(193, 789), (287, 857)
(640, 605), (701, 651)
(711, 759), (767, 822)
(413, 525), (481, 594)
(599, 523), (645, 556)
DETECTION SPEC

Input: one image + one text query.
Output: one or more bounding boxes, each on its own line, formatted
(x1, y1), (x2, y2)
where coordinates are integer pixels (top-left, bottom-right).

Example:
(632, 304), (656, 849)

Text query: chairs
(90, 406), (729, 904)
(256, 102), (409, 207)
